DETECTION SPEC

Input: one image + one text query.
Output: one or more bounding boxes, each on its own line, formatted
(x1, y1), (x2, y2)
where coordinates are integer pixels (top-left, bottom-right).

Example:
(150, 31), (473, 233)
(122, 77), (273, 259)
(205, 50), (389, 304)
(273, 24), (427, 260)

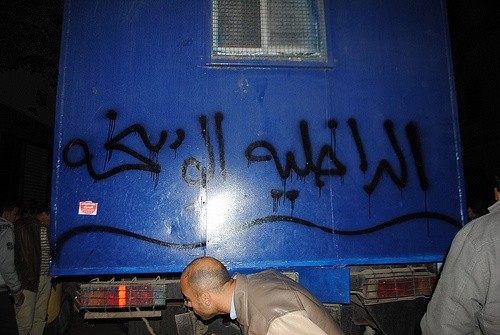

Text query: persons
(0, 203), (62, 335)
(419, 175), (500, 335)
(180, 256), (346, 335)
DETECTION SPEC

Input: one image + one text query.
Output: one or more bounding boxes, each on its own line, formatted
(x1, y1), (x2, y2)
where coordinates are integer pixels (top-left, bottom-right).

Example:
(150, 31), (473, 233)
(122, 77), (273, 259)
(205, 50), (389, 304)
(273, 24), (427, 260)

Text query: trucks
(47, 0), (467, 334)
